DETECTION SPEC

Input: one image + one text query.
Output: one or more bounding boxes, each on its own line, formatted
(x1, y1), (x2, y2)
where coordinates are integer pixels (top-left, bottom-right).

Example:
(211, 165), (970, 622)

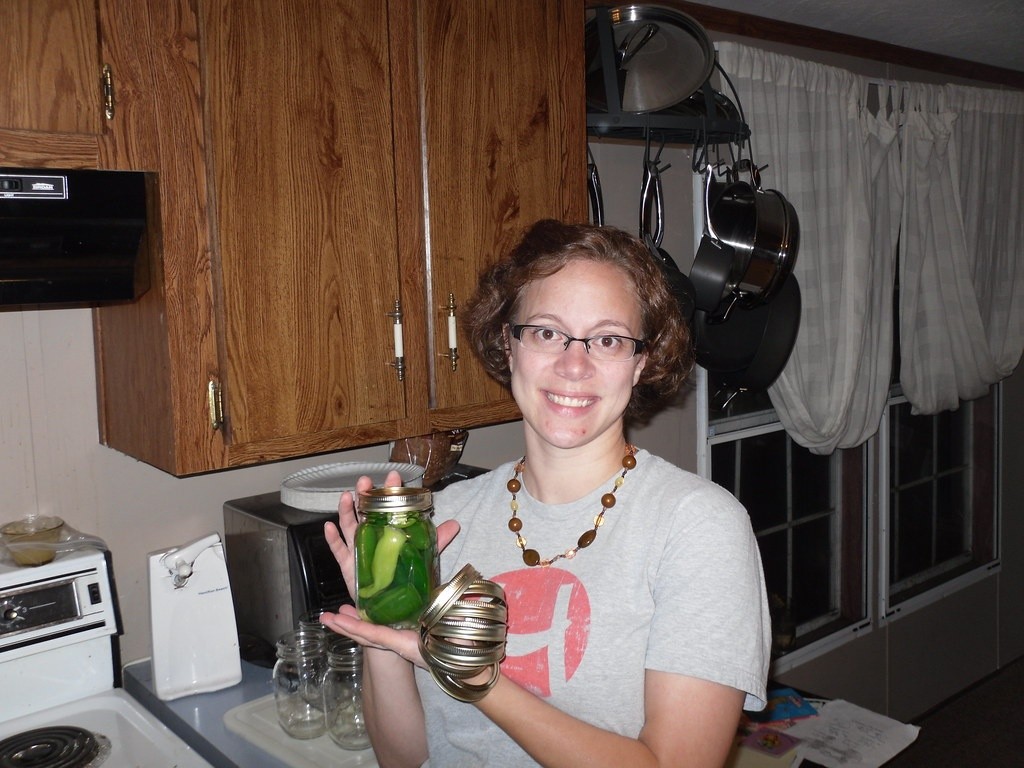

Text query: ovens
(221, 461), (494, 673)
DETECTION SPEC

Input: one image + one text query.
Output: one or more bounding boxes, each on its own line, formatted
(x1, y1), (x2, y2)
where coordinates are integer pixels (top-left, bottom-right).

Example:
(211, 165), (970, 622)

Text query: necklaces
(507, 444), (637, 567)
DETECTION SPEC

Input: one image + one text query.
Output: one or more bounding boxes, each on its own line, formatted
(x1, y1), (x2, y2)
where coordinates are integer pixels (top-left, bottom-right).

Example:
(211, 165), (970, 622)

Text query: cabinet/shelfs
(0, 0), (589, 481)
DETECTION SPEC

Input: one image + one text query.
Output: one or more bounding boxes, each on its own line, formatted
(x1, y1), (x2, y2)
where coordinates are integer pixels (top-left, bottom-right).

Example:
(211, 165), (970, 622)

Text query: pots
(582, 144), (801, 412)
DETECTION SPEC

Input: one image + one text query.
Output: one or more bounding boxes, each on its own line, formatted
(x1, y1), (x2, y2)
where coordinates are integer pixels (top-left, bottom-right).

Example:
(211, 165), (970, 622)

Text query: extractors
(0, 168), (156, 312)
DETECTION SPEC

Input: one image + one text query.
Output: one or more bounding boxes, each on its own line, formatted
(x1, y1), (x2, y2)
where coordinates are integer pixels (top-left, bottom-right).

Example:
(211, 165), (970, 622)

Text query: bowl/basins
(0, 516), (66, 569)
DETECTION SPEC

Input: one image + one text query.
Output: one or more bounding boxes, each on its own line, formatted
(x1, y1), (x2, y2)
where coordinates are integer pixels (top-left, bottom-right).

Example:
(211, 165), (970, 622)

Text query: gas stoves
(0, 532), (215, 768)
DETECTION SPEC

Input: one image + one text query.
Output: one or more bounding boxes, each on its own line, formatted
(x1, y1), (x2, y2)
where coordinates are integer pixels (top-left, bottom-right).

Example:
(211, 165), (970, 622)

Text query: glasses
(508, 321), (646, 361)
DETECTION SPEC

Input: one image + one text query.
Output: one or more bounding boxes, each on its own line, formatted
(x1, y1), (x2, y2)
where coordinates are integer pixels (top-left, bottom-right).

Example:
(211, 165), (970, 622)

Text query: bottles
(273, 628), (330, 741)
(321, 632), (385, 751)
(354, 488), (440, 631)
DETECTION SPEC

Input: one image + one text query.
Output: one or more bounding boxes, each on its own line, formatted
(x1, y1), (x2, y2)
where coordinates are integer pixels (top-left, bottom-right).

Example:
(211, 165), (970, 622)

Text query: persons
(318, 218), (772, 767)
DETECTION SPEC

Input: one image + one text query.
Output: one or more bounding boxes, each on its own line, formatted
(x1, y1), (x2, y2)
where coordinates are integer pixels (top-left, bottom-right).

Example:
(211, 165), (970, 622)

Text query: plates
(279, 461), (426, 513)
